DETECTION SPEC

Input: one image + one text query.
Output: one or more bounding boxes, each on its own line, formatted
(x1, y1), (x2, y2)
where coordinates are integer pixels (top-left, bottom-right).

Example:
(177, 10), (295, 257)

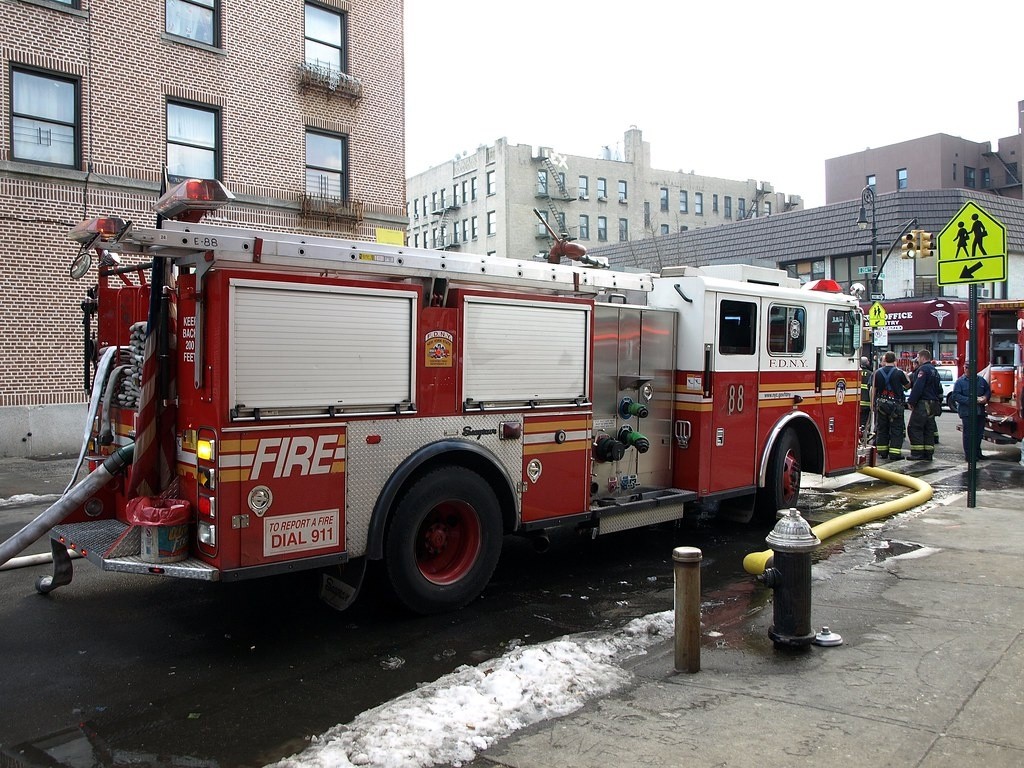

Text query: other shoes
(907, 455), (932, 460)
(978, 454), (989, 460)
(882, 455), (888, 459)
(890, 456), (905, 460)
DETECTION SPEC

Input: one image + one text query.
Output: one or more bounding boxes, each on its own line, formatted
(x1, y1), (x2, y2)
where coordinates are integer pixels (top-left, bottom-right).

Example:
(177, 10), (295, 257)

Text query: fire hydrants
(765, 510), (820, 656)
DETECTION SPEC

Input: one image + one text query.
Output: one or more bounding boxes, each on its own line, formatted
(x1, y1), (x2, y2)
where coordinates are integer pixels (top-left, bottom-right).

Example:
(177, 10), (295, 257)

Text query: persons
(860, 349), (944, 461)
(953, 361), (991, 461)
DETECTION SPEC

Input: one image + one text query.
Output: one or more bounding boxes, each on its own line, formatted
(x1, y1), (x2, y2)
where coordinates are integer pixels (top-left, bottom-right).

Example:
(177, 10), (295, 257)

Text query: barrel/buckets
(990, 367), (1014, 397)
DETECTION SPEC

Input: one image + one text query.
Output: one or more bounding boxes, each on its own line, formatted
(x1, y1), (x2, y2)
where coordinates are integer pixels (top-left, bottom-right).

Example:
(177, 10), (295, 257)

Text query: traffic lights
(901, 232), (917, 260)
(919, 232), (933, 258)
(862, 329), (870, 341)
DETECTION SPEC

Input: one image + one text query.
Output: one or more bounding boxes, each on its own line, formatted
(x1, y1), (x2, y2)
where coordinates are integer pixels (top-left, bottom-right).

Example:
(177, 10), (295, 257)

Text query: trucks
(955, 297), (1024, 445)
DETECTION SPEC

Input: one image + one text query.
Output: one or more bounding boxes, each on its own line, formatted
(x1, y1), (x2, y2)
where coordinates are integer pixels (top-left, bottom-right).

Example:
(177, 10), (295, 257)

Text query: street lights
(855, 184), (880, 394)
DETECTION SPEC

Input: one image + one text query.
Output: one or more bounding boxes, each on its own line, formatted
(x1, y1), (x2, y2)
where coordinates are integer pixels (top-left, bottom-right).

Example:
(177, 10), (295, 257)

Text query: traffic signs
(870, 292), (886, 302)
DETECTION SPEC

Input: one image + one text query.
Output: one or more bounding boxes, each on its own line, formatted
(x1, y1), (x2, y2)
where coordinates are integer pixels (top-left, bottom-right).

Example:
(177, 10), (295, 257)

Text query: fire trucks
(52, 179), (862, 613)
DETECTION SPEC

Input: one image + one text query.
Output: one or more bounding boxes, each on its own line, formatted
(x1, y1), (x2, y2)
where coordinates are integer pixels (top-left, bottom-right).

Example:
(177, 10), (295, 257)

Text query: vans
(904, 365), (961, 413)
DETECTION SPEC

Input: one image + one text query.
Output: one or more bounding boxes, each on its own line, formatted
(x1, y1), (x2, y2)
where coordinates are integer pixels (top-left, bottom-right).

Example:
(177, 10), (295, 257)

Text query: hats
(860, 356), (869, 368)
(911, 357), (919, 363)
(963, 362), (970, 368)
